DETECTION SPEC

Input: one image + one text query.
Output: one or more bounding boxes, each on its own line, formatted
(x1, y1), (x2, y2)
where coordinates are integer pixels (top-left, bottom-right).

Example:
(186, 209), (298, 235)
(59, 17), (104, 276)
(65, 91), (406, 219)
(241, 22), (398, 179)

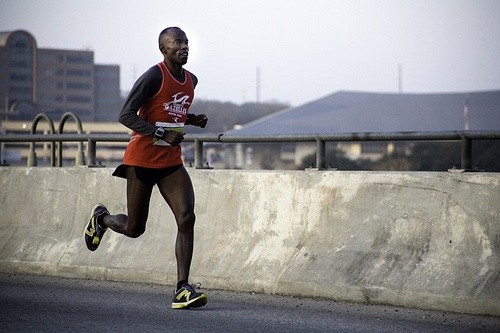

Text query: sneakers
(83, 203), (110, 253)
(172, 282), (207, 310)
